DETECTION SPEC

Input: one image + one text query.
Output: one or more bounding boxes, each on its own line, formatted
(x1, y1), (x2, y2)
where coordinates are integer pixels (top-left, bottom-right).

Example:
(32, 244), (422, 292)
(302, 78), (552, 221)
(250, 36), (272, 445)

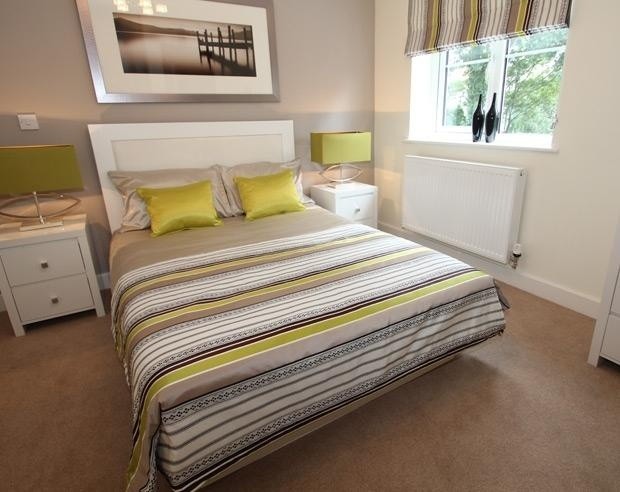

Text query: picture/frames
(75, 0), (281, 104)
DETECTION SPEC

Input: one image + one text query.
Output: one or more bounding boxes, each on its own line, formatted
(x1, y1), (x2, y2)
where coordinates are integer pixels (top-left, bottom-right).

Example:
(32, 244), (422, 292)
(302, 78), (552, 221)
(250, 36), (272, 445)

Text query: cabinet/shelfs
(0, 214), (106, 338)
(587, 217), (620, 368)
(311, 180), (378, 229)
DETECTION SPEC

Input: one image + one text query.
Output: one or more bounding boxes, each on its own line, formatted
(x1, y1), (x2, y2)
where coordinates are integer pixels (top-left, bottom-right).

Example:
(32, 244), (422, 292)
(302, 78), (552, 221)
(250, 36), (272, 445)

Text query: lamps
(311, 130), (372, 188)
(0, 144), (85, 231)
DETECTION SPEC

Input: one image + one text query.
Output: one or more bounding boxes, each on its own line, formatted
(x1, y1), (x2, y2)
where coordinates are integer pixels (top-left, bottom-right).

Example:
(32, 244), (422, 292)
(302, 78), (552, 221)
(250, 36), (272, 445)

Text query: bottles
(485, 93), (497, 143)
(473, 94), (484, 142)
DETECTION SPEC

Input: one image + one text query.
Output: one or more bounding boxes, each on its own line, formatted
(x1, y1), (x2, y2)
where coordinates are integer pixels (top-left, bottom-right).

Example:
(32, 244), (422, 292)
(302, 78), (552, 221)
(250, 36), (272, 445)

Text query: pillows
(107, 166), (236, 233)
(212, 157), (316, 216)
(135, 179), (223, 238)
(233, 169), (306, 221)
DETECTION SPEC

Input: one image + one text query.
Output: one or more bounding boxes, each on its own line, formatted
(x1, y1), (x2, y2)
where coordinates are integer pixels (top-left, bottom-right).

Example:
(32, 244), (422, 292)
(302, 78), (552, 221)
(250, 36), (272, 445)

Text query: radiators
(402, 154), (527, 264)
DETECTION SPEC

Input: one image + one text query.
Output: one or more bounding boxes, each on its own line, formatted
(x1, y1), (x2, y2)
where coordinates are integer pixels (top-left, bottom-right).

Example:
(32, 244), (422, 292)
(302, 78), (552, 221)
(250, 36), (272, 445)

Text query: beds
(87, 120), (512, 492)
